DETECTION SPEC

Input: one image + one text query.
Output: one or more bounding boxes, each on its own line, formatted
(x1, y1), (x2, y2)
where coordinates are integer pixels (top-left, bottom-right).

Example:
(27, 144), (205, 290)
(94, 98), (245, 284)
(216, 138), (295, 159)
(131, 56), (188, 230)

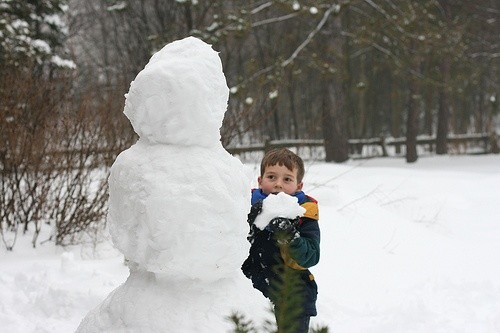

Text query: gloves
(247, 200), (263, 234)
(268, 215), (300, 242)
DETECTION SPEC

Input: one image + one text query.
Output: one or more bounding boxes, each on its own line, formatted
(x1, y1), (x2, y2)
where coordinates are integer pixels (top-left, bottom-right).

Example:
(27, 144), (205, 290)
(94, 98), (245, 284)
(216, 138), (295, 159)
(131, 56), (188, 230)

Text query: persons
(242, 149), (320, 333)
